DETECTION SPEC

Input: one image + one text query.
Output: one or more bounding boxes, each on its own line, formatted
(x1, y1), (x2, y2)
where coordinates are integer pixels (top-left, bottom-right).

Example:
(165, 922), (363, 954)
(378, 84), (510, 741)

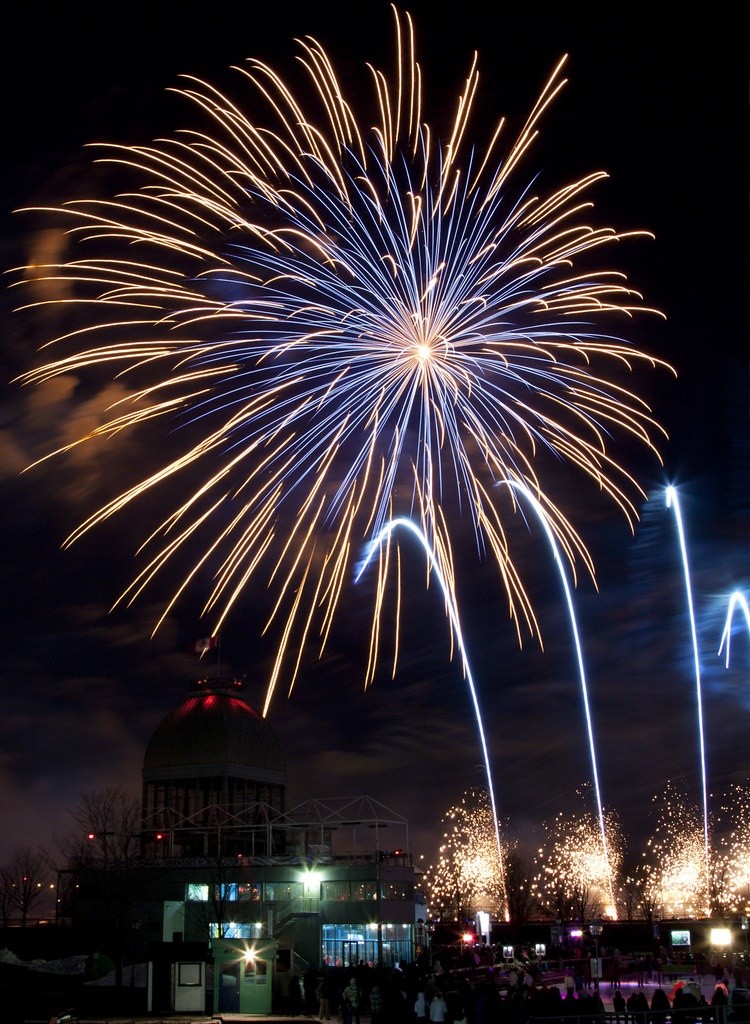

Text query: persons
(289, 943), (750, 1024)
